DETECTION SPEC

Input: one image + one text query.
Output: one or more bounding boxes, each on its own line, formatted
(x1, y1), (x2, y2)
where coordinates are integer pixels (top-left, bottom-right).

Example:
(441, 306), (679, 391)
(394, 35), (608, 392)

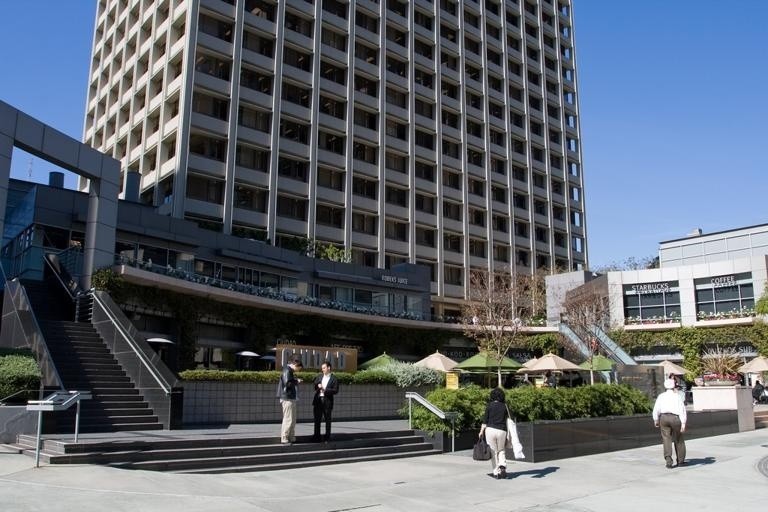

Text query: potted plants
(694, 344), (747, 386)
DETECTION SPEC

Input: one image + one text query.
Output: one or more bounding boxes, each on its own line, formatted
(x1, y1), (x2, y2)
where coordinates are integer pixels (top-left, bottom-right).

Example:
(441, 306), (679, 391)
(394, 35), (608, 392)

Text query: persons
(276, 359), (303, 444)
(653, 378), (688, 468)
(751, 380), (763, 401)
(665, 372), (678, 390)
(478, 388), (509, 478)
(309, 361), (338, 443)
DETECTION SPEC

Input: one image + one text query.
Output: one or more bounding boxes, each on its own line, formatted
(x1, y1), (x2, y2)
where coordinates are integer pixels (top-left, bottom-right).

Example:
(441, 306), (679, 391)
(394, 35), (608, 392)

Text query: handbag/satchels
(473, 435), (491, 460)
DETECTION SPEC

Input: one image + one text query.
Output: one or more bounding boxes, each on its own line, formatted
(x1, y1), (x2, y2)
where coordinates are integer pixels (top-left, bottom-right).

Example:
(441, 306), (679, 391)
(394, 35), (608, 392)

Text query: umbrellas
(356, 349), (617, 391)
(657, 360), (690, 379)
(737, 354), (768, 386)
